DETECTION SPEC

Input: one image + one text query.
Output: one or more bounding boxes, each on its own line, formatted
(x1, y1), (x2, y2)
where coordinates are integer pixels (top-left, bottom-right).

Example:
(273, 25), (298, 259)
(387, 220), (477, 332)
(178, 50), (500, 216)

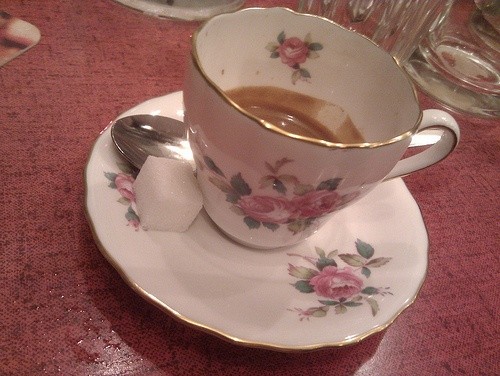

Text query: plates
(85, 89), (429, 350)
(111, 0), (248, 22)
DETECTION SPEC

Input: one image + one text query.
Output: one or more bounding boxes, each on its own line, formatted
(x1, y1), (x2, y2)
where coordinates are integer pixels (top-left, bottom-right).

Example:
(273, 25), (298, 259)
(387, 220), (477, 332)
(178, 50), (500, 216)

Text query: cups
(419, 0), (500, 96)
(296, 0), (456, 69)
(186, 6), (461, 252)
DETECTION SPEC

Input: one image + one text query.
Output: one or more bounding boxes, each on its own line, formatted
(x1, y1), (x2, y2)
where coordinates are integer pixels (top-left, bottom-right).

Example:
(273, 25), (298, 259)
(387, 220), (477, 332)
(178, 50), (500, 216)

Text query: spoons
(110, 114), (198, 177)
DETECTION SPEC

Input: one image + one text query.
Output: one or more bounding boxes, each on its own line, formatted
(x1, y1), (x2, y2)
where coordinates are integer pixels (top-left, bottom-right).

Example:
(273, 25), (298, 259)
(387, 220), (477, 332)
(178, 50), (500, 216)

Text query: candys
(133, 155), (204, 232)
